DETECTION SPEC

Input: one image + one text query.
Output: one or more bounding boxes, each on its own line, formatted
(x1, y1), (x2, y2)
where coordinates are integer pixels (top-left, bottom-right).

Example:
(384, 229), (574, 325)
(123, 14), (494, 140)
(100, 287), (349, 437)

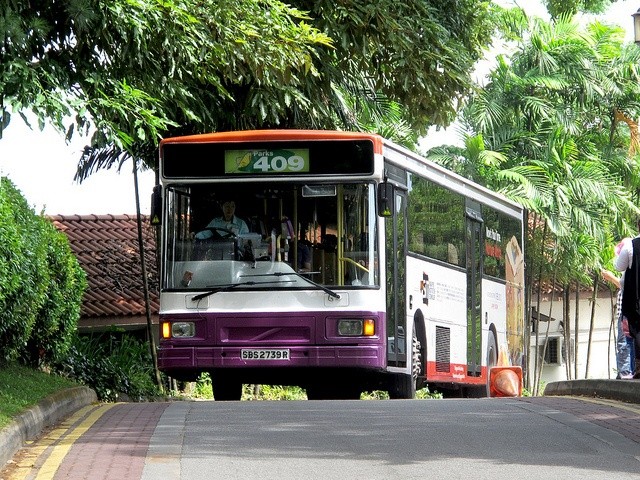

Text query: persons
(601, 269), (631, 379)
(269, 215), (294, 262)
(194, 199), (250, 261)
(615, 217), (640, 379)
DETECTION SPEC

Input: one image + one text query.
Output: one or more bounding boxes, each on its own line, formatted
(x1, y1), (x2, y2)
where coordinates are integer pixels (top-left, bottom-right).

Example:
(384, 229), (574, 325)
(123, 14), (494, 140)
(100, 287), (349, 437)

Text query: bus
(155, 128), (529, 401)
